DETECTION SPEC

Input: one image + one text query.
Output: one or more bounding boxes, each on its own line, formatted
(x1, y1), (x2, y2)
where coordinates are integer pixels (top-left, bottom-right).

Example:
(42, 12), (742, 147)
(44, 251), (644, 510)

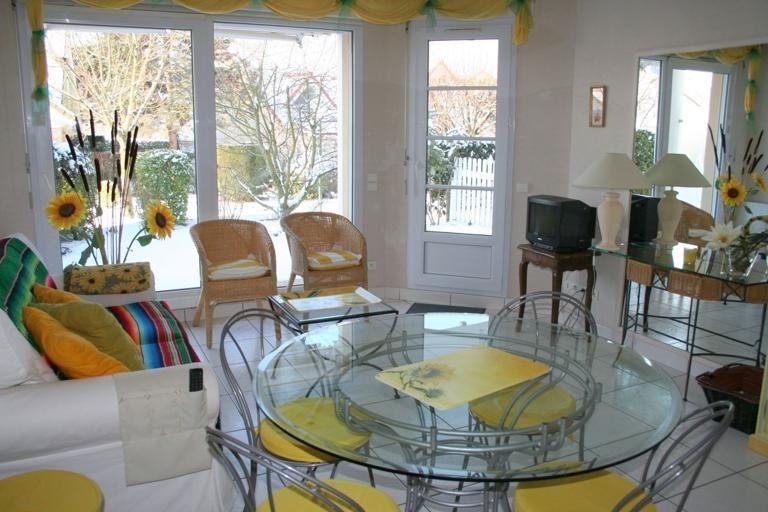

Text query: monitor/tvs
(628, 193), (661, 243)
(525, 194), (597, 255)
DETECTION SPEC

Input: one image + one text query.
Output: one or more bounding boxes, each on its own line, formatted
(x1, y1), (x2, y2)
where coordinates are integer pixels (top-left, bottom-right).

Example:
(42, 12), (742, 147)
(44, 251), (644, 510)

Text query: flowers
(706, 122), (766, 226)
(44, 105), (175, 266)
(700, 219), (768, 263)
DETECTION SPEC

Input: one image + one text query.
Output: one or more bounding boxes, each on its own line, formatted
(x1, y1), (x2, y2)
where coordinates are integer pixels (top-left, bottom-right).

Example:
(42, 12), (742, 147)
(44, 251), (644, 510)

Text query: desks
(251, 312), (684, 512)
(516, 244), (600, 349)
(588, 240), (767, 401)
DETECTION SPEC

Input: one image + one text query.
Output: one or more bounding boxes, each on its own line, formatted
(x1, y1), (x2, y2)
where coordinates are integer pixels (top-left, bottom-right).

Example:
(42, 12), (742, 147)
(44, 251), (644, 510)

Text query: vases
(719, 244), (760, 279)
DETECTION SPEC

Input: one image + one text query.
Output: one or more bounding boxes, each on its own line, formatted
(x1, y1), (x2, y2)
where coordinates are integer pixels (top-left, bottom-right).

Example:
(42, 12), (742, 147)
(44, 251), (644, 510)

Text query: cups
(683, 246), (697, 267)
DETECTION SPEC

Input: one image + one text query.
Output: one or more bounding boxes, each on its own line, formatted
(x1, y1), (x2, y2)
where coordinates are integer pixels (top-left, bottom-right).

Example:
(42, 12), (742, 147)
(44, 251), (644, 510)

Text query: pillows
(24, 279), (145, 377)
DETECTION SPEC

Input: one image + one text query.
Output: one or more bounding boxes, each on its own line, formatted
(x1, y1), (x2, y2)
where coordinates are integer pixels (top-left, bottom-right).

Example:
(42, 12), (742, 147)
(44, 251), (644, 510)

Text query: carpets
(404, 303), (485, 315)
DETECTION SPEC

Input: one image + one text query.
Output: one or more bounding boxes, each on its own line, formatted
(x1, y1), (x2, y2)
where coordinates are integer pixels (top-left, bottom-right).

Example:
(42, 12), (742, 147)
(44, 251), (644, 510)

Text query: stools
(0, 469), (105, 512)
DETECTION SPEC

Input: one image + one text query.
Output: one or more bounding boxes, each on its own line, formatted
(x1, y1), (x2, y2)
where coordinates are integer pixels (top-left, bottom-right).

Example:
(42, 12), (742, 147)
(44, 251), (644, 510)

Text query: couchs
(0, 232), (233, 512)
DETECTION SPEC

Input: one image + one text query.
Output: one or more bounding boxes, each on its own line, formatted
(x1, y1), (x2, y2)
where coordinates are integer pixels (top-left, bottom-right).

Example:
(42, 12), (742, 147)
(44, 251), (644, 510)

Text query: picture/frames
(588, 85), (607, 127)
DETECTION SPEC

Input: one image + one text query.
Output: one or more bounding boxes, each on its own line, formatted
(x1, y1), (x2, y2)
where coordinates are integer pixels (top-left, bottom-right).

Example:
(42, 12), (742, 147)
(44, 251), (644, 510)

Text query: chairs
(279, 212), (370, 334)
(206, 425), (399, 512)
(219, 309), (375, 512)
(452, 291), (603, 510)
(188, 218), (282, 350)
(510, 400), (736, 512)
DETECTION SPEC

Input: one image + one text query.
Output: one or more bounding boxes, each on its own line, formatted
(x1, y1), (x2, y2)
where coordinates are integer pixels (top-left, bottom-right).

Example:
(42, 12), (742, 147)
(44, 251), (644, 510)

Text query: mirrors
(616, 44), (768, 371)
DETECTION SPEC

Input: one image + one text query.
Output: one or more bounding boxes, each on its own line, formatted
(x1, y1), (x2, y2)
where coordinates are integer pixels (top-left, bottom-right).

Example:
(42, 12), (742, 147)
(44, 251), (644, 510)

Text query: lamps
(571, 152), (652, 250)
(644, 154), (713, 246)
